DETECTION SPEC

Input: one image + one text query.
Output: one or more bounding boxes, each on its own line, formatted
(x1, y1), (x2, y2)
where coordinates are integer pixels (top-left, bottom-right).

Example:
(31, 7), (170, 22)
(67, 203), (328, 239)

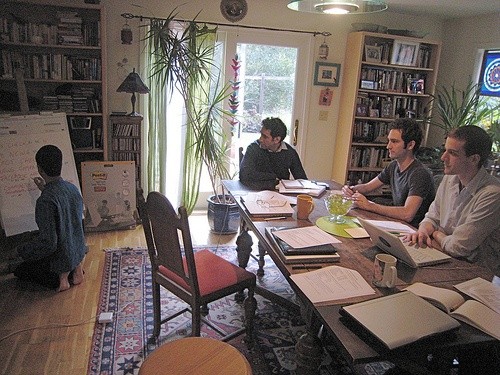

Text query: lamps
(287, 0), (388, 15)
(116, 67), (149, 116)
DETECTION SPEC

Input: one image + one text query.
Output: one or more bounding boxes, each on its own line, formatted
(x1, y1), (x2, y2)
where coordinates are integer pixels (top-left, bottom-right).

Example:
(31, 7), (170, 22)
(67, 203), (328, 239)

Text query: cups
(372, 253), (398, 289)
(296, 195), (314, 219)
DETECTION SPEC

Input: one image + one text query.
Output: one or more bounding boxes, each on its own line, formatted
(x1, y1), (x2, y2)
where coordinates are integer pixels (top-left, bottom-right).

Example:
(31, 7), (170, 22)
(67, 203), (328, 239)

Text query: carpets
(85, 245), (395, 375)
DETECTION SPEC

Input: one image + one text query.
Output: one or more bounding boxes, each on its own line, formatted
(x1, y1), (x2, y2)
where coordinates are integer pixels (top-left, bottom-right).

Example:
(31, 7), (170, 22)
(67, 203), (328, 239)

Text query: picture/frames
(219, 0), (248, 22)
(314, 61), (341, 88)
(390, 40), (420, 67)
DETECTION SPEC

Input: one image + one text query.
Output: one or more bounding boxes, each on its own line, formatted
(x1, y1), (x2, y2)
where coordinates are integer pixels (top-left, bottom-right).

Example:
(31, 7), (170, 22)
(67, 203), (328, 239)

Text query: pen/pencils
(292, 266), (322, 269)
(264, 216), (287, 220)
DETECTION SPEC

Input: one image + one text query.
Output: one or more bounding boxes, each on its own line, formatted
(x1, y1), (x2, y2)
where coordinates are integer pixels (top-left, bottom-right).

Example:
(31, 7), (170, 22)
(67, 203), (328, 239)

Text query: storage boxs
(81, 161), (137, 232)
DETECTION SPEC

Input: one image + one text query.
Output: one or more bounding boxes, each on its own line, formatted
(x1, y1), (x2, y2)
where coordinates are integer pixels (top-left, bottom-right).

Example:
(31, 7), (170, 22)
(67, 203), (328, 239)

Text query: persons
(402, 125), (500, 273)
(342, 119), (436, 229)
(14, 145), (86, 291)
(240, 116), (308, 190)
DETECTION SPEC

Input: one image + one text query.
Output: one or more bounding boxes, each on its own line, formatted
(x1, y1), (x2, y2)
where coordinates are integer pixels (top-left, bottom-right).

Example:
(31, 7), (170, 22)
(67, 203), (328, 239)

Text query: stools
(138, 337), (253, 375)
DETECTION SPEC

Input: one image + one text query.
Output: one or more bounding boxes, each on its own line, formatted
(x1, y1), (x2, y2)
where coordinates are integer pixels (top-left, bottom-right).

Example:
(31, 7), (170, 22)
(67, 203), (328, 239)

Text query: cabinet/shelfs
(110, 114), (144, 192)
(0, 0), (107, 162)
(330, 32), (443, 187)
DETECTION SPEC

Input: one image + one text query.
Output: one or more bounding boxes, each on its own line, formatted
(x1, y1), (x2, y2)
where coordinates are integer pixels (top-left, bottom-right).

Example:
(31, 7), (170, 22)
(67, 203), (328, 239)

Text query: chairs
(134, 188), (257, 337)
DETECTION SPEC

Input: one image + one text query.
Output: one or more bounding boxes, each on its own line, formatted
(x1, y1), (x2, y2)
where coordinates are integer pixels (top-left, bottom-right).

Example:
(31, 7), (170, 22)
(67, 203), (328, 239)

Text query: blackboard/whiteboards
(0, 111), (86, 237)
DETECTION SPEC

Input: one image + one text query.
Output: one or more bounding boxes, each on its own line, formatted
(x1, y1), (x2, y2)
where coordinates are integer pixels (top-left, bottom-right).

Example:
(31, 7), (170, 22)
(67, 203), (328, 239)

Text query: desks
(221, 179), (500, 375)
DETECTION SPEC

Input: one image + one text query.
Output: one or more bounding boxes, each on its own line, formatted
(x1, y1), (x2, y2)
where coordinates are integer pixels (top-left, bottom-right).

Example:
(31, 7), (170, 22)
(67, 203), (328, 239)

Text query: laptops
(357, 216), (452, 268)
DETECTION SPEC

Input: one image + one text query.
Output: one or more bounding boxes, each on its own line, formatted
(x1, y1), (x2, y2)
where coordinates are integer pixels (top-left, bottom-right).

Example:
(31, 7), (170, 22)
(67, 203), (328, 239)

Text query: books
(356, 93), (423, 119)
(339, 290), (462, 349)
(112, 124), (140, 209)
(240, 191), (294, 221)
(351, 120), (397, 144)
(93, 121), (104, 150)
(0, 49), (102, 80)
(0, 9), (101, 46)
(359, 65), (427, 94)
(346, 171), (393, 195)
(0, 84), (102, 113)
(264, 225), (341, 263)
(402, 281), (500, 341)
(365, 40), (431, 68)
(348, 146), (395, 169)
(279, 179), (326, 197)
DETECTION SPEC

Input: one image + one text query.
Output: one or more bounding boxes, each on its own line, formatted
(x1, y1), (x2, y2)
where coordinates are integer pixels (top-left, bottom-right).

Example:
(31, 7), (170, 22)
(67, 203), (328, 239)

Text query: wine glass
(325, 196), (354, 223)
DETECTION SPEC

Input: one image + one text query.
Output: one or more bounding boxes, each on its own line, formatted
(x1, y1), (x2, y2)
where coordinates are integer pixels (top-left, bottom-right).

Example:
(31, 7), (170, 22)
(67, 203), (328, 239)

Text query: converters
(99, 313), (114, 323)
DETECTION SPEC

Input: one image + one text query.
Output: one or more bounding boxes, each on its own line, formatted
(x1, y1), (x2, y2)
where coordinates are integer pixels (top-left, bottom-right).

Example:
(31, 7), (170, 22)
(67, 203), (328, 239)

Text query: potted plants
(134, 2), (246, 235)
